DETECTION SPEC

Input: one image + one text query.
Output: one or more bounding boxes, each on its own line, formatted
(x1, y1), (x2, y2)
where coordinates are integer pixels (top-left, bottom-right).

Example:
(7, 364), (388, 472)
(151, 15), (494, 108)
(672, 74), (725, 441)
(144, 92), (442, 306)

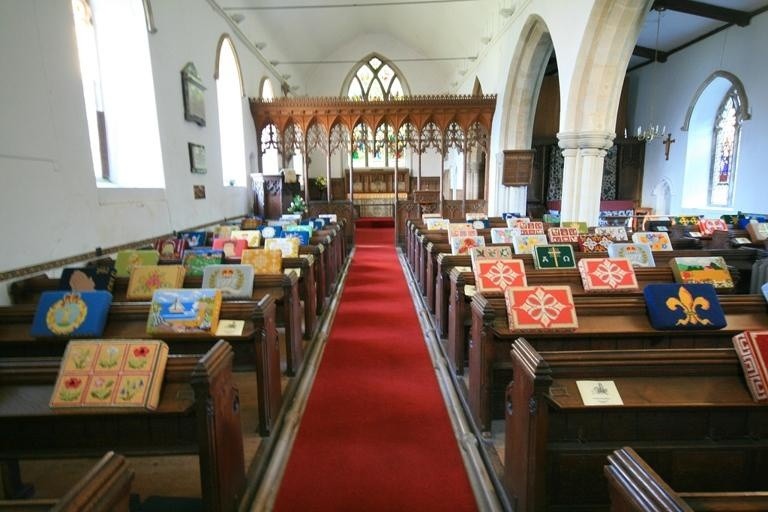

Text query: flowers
(288, 194), (309, 213)
(315, 175), (327, 190)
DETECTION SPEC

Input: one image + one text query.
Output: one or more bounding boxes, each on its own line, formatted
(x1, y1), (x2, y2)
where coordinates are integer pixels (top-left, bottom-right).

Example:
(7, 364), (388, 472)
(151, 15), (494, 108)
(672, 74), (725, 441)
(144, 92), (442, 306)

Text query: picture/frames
(189, 143), (207, 174)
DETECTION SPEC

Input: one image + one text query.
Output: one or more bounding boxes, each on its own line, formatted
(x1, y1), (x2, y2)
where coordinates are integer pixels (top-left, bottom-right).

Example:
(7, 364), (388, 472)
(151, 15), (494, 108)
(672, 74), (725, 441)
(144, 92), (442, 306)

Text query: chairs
(2, 447), (136, 511)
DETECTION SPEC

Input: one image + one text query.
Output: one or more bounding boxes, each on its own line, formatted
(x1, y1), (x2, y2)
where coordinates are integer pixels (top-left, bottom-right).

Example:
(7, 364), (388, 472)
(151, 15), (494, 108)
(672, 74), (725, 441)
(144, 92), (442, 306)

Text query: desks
(505, 336), (768, 510)
(405, 213), (766, 433)
(347, 192), (407, 218)
(2, 339), (248, 511)
(2, 216), (347, 436)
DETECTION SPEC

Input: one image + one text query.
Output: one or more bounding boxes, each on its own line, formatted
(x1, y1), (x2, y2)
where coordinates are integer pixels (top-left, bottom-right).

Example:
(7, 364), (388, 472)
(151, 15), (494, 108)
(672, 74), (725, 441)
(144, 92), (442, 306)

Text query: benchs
(603, 445), (766, 511)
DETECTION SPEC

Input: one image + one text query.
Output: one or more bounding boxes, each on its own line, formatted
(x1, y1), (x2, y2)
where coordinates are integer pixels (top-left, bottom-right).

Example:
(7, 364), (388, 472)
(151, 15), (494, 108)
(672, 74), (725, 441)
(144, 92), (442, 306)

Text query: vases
(315, 190), (328, 200)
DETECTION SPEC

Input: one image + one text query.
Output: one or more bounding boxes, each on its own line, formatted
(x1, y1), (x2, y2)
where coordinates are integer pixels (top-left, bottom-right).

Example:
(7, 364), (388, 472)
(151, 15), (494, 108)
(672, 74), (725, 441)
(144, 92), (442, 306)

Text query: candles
(624, 125), (666, 139)
